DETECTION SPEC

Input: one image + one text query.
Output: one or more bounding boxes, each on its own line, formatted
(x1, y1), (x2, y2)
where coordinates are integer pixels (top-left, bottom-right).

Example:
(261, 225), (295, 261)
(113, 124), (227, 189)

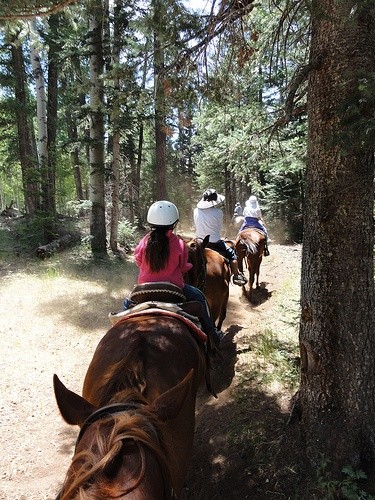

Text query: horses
(51, 369), (196, 500)
(229, 226), (268, 296)
(232, 216), (247, 238)
(78, 232), (213, 481)
(201, 237), (242, 327)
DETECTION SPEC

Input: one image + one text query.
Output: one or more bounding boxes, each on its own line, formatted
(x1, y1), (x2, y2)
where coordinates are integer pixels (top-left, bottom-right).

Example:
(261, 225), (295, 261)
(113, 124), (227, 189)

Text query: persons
(239, 195), (270, 257)
(229, 202), (244, 229)
(123, 200), (225, 357)
(193, 189), (248, 287)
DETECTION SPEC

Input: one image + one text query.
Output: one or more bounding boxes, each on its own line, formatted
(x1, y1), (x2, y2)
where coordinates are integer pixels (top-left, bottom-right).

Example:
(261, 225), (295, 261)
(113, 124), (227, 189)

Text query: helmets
(146, 201), (179, 225)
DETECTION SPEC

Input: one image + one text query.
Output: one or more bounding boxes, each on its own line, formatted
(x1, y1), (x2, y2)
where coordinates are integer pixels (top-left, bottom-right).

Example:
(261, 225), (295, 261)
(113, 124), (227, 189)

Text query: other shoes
(264, 249), (269, 256)
(212, 328), (224, 342)
(239, 272), (248, 282)
(233, 275), (245, 285)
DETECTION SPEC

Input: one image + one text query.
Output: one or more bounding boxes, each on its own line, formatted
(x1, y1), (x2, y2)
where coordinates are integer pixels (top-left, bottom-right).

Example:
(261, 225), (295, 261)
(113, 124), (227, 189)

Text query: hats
(245, 196), (259, 210)
(197, 188), (226, 209)
